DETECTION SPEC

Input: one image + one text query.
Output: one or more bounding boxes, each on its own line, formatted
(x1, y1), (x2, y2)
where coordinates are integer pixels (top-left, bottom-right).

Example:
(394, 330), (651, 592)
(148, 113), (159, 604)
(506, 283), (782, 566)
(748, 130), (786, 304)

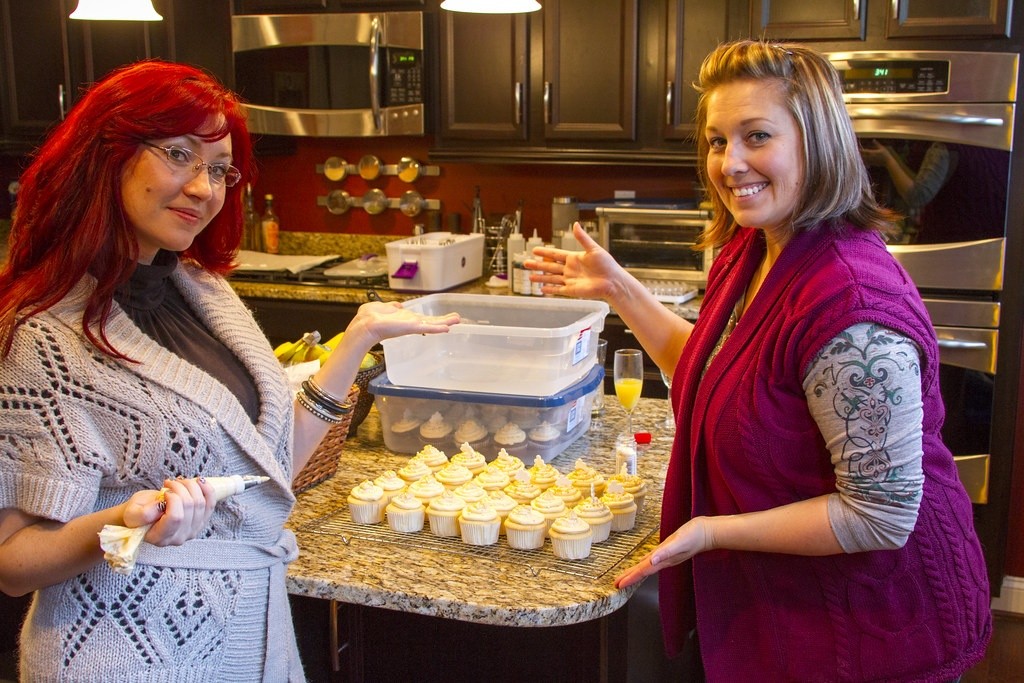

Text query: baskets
(290, 386), (362, 494)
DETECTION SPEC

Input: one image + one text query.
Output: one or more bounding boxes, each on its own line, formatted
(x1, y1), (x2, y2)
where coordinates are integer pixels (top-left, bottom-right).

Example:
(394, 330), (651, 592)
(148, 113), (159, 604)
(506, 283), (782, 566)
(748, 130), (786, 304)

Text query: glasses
(139, 140), (242, 188)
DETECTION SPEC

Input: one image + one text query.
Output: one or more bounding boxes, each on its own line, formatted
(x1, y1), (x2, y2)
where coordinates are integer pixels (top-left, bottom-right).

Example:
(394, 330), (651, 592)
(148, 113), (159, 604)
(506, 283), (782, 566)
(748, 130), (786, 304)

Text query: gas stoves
(223, 260), (391, 290)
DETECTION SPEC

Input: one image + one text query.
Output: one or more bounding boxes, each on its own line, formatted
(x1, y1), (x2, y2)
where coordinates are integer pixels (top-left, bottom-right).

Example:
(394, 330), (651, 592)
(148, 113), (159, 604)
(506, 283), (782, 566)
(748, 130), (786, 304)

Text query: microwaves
(592, 205), (722, 291)
(230, 12), (425, 135)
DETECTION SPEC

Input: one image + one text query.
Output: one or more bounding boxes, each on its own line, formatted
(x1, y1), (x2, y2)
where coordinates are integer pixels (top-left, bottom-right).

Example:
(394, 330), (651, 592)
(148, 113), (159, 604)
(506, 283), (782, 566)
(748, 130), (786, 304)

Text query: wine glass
(613, 348), (643, 440)
(660, 369), (674, 429)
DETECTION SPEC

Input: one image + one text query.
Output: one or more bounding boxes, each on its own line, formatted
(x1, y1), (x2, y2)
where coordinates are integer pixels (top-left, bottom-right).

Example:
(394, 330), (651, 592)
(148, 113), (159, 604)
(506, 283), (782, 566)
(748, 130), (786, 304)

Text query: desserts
(347, 442), (648, 560)
(389, 398), (575, 462)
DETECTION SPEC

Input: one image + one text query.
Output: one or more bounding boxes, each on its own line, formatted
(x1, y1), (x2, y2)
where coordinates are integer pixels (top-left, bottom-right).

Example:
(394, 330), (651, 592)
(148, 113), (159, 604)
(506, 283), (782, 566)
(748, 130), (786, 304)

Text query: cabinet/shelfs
(0, 0), (233, 160)
(428, 0), (1023, 168)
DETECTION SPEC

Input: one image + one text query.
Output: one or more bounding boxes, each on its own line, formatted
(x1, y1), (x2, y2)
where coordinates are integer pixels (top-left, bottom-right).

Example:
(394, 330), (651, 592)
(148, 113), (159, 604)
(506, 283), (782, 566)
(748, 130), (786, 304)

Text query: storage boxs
(385, 231), (484, 292)
(368, 293), (610, 465)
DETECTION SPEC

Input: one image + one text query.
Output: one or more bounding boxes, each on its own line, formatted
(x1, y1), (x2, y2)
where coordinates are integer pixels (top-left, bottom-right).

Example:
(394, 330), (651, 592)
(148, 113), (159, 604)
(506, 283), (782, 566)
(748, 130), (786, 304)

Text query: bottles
(551, 196), (579, 231)
(511, 252), (531, 296)
(531, 254), (544, 296)
(239, 182), (261, 252)
(261, 195), (279, 254)
(506, 225), (525, 290)
(589, 225), (599, 245)
(577, 225), (586, 252)
(552, 231), (565, 249)
(562, 222), (577, 251)
(526, 228), (544, 251)
(615, 433), (638, 476)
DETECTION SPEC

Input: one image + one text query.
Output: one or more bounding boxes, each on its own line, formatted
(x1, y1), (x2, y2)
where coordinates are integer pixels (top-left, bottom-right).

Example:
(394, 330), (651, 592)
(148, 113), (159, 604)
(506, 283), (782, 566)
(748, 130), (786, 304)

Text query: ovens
(822, 49), (1021, 507)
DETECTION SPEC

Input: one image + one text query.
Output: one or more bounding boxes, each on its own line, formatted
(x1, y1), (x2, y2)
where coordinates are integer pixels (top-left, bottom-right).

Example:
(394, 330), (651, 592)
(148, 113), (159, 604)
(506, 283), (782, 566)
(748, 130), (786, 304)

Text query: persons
(0, 59), (460, 683)
(524, 42), (994, 683)
(859, 140), (1010, 244)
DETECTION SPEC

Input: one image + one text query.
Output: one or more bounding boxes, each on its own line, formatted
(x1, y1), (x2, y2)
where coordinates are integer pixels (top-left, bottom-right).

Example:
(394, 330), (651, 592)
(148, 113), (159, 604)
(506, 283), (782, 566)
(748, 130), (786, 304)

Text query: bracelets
(296, 375), (352, 424)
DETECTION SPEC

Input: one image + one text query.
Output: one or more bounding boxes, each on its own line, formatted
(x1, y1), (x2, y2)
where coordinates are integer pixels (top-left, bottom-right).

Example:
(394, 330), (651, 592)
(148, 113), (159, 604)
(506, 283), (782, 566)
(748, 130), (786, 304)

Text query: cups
(591, 338), (609, 417)
